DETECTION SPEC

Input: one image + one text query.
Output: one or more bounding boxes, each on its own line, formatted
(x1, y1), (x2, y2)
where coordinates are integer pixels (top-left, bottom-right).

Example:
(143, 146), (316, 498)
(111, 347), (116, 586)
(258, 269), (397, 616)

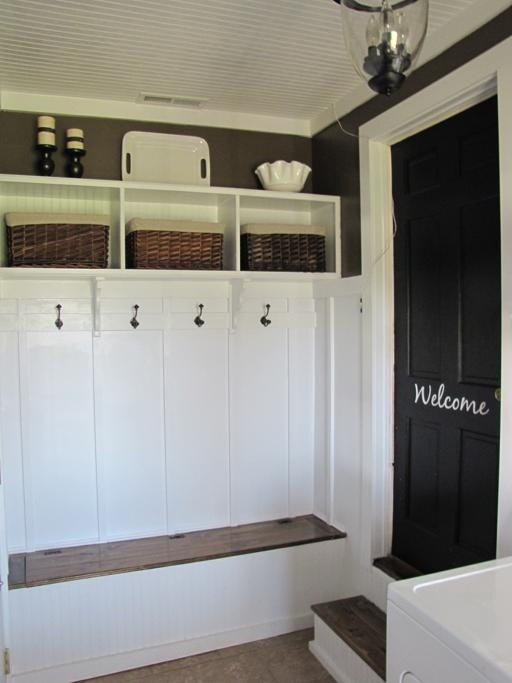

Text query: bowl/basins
(254, 160), (312, 192)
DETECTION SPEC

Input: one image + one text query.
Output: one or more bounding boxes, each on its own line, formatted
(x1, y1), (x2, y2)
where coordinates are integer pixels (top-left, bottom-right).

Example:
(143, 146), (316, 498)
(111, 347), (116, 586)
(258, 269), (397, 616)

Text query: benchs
(1, 515), (351, 682)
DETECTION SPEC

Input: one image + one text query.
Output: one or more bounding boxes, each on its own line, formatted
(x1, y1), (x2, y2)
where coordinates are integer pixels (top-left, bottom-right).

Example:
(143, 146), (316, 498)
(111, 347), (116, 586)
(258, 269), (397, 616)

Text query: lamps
(334, 0), (429, 95)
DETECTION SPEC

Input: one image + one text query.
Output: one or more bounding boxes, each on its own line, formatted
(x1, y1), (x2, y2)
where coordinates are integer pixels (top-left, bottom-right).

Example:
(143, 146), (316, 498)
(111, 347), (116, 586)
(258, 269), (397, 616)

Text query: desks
(385, 554), (511, 683)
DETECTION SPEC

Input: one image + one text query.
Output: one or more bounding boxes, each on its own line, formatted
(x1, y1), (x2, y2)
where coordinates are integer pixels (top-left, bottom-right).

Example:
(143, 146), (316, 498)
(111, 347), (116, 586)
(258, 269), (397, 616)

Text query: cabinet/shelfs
(0, 174), (343, 280)
